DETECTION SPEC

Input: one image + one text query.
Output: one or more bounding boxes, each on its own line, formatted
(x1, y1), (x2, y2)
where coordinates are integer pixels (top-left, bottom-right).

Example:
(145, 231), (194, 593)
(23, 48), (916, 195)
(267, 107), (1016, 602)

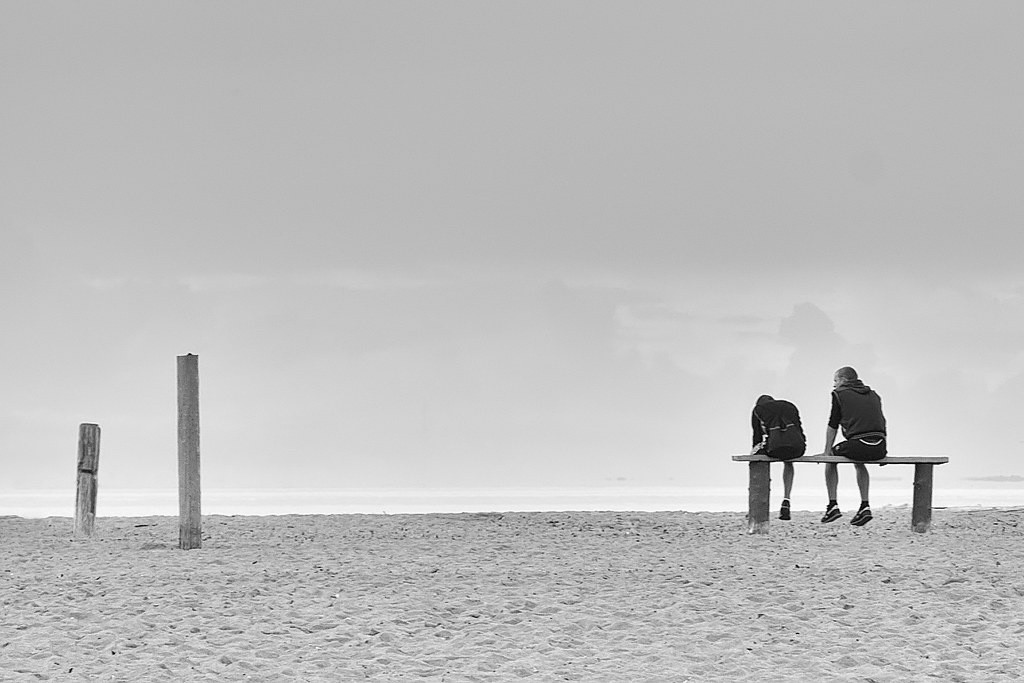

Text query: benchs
(732, 454), (949, 535)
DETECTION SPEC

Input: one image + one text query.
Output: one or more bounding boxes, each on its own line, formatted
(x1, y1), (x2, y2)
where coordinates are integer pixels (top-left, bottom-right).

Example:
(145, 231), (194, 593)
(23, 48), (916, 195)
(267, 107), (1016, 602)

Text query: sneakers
(851, 507), (873, 525)
(821, 505), (842, 522)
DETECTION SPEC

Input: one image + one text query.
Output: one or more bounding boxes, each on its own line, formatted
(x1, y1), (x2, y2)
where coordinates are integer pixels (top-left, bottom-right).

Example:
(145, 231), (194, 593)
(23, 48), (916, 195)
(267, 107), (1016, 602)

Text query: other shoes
(779, 499), (791, 520)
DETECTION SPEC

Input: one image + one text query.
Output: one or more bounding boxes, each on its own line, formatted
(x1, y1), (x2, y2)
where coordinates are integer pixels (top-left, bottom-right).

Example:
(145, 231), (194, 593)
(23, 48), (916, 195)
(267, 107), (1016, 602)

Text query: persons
(745, 394), (807, 521)
(813, 366), (888, 527)
